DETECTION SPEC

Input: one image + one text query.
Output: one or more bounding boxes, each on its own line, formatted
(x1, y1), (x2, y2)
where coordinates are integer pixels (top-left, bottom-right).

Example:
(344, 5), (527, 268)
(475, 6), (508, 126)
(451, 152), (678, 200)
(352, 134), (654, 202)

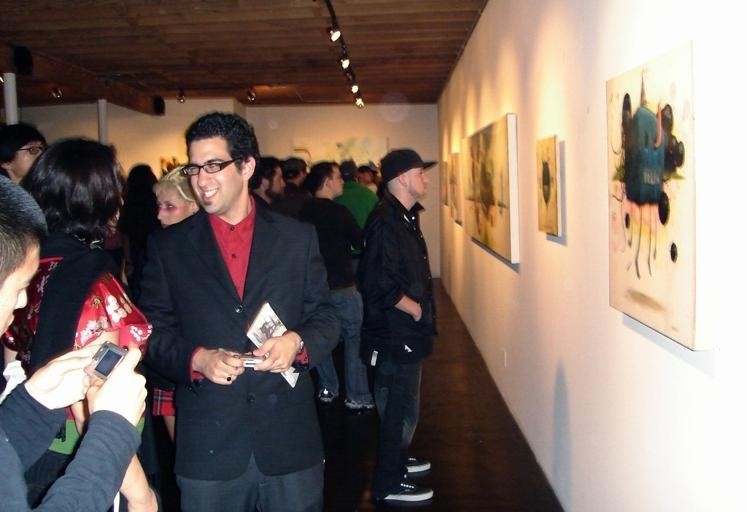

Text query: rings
(226, 374), (232, 383)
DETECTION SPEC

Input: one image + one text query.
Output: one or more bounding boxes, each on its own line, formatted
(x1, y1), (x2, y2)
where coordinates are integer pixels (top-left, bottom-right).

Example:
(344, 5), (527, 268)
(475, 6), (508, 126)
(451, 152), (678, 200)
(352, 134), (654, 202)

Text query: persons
(360, 147), (442, 504)
(1, 121), (48, 185)
(153, 165), (199, 228)
(134, 107), (342, 509)
(0, 176), (151, 511)
(303, 161), (377, 410)
(250, 157), (380, 226)
(120, 164), (163, 239)
(16, 136), (169, 510)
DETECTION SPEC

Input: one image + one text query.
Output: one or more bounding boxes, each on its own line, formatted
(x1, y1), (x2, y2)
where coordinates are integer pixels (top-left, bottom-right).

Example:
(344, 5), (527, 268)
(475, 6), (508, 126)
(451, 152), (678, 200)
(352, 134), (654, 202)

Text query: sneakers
(405, 456), (431, 472)
(384, 481), (434, 501)
(319, 388), (376, 408)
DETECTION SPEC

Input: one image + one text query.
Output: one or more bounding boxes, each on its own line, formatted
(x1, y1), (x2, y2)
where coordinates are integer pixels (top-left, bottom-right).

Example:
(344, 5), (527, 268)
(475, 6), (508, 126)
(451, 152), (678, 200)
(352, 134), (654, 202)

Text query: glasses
(18, 144), (44, 154)
(182, 158), (241, 176)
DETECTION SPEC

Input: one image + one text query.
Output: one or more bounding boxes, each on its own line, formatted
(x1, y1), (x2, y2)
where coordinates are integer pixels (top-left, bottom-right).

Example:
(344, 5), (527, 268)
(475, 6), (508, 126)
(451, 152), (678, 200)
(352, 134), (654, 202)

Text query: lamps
(246, 85), (258, 103)
(174, 84), (187, 105)
(320, 0), (364, 112)
(48, 85), (65, 102)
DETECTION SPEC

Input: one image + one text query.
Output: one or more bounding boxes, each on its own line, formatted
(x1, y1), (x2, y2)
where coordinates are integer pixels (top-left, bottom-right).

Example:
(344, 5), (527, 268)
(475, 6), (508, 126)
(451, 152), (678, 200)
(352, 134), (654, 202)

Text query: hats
(381, 150), (435, 183)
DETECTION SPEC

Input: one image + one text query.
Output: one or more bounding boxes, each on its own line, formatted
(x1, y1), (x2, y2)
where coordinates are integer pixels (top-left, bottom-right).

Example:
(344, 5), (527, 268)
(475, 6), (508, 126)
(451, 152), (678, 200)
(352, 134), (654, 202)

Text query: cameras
(237, 354), (266, 367)
(83, 342), (127, 383)
